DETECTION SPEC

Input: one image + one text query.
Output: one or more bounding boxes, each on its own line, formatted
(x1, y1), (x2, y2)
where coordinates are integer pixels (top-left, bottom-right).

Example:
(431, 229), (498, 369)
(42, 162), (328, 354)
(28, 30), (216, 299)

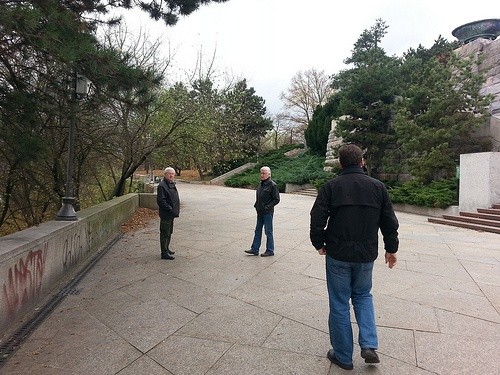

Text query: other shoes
(325, 348), (354, 369)
(168, 249), (175, 255)
(361, 345), (380, 364)
(245, 248), (258, 255)
(260, 248), (275, 257)
(161, 253), (174, 260)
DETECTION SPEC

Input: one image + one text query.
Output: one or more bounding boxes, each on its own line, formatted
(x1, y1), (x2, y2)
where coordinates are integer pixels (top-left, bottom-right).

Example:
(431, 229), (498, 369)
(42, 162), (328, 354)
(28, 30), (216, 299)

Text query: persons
(155, 167), (180, 259)
(244, 167), (280, 257)
(309, 144), (400, 370)
